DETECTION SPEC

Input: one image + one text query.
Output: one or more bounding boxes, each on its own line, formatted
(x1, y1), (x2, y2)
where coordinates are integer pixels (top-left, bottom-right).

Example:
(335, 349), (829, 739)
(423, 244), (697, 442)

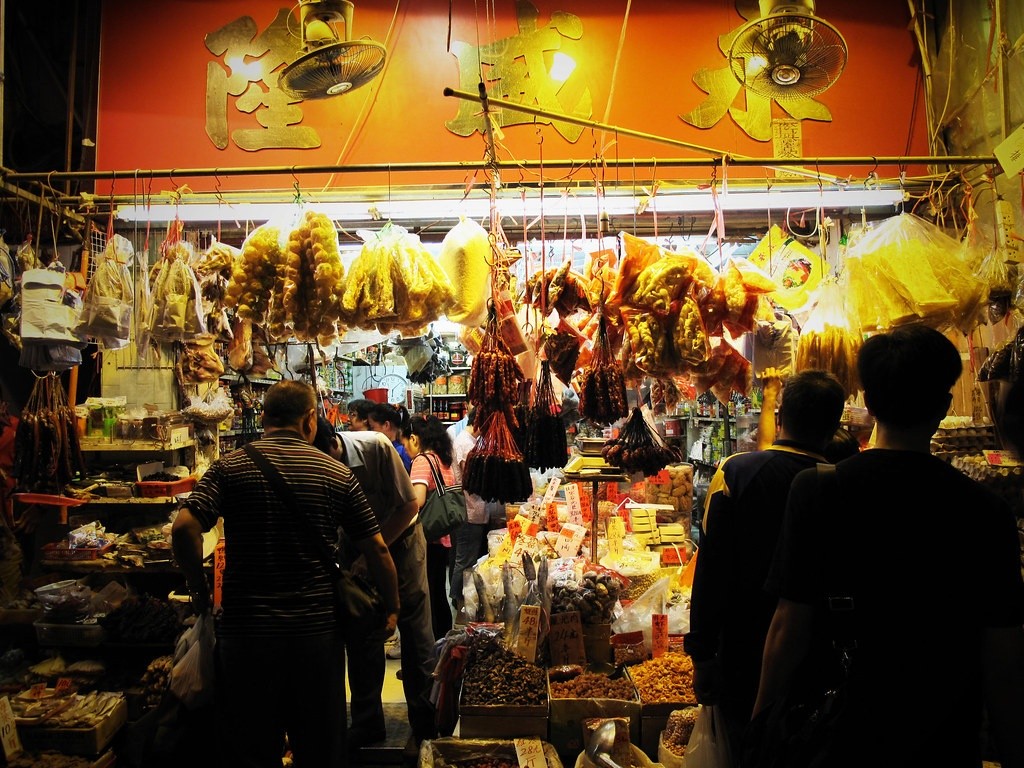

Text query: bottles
(438, 400), (444, 421)
(443, 401), (450, 422)
(432, 400), (437, 420)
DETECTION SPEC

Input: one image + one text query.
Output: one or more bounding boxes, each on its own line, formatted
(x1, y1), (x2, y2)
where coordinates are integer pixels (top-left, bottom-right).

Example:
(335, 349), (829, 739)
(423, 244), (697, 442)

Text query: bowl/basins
(34, 580), (80, 601)
(147, 541), (171, 560)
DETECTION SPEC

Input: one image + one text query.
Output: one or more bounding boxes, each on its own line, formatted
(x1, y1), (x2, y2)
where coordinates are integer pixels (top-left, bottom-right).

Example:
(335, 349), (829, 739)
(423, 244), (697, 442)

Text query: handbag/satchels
(332, 535), (401, 646)
(417, 483), (468, 543)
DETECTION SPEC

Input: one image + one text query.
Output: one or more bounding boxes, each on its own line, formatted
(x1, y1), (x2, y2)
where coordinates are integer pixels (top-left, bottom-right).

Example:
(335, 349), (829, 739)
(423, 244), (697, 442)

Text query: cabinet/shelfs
(430, 367), (472, 426)
(101, 343), (372, 463)
(43, 436), (206, 646)
(667, 415), (736, 529)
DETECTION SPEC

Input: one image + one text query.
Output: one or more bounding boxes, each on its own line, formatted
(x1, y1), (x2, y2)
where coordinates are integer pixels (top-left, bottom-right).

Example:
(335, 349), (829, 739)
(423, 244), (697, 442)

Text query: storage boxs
(136, 479), (196, 498)
(458, 662), (698, 756)
(419, 739), (563, 768)
(20, 700), (128, 754)
(487, 528), (508, 552)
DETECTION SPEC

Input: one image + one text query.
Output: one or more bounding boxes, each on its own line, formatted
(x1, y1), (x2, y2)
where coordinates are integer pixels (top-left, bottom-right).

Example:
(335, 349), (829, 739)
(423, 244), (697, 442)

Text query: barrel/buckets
(362, 375), (389, 404)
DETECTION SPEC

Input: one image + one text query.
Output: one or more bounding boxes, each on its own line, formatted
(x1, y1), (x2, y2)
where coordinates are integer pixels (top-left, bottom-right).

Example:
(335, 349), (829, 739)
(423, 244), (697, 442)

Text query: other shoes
(452, 595), (465, 610)
(347, 725), (387, 747)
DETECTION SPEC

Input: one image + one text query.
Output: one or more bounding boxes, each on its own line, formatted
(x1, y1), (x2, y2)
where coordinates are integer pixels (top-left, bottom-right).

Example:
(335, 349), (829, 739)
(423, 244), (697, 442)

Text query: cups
(103, 418), (117, 439)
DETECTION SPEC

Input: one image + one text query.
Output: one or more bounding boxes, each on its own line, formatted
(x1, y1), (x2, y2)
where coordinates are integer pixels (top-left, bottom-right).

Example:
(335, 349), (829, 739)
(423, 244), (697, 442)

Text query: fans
(276, 0), (387, 100)
(729, 0), (850, 101)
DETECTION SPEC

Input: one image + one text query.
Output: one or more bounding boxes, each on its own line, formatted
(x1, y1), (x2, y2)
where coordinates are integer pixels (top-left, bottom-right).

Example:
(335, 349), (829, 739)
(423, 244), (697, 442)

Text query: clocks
(377, 374), (408, 405)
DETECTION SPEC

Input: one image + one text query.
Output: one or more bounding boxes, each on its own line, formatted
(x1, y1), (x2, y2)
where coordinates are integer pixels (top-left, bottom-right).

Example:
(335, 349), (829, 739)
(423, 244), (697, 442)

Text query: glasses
(348, 411), (359, 417)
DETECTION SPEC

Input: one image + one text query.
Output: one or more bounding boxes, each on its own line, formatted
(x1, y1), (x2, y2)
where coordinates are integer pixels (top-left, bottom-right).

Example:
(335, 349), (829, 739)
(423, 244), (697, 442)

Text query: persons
(689, 324), (1023, 768)
(171, 380), (532, 768)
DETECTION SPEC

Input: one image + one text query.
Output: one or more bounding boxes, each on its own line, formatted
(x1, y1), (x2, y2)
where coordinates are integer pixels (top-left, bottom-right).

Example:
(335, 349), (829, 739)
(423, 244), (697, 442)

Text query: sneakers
(405, 733), (418, 756)
(386, 639), (401, 659)
